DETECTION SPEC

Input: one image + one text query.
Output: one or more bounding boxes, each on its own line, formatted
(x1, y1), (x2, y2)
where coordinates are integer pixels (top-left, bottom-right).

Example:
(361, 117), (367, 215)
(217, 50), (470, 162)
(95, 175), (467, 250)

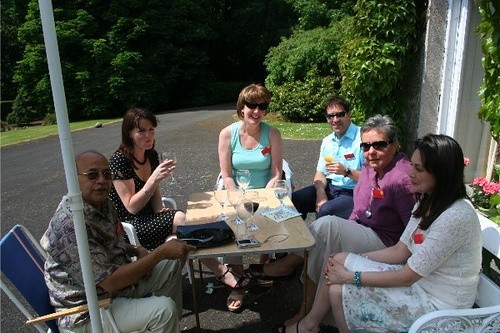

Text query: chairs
(216, 159), (293, 265)
(121, 197), (192, 288)
(0, 224), (122, 333)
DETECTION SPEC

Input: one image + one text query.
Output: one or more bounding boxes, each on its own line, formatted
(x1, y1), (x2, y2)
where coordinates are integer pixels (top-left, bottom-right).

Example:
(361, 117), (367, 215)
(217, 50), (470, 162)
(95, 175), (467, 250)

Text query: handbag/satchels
(176, 221), (235, 248)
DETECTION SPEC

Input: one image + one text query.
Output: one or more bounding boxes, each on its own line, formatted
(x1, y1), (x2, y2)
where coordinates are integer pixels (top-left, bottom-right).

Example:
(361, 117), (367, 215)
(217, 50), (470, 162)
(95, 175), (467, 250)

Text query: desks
(185, 187), (316, 333)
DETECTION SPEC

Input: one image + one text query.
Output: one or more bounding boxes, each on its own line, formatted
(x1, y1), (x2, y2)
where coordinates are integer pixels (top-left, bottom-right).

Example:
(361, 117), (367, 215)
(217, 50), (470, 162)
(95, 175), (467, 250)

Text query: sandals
(274, 320), (324, 333)
(226, 290), (246, 311)
(218, 269), (251, 289)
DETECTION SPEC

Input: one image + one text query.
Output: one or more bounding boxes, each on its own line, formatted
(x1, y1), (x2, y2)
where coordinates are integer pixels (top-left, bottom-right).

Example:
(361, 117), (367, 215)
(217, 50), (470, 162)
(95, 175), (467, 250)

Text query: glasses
(360, 140), (392, 152)
(326, 111), (346, 119)
(244, 99), (268, 110)
(78, 171), (116, 180)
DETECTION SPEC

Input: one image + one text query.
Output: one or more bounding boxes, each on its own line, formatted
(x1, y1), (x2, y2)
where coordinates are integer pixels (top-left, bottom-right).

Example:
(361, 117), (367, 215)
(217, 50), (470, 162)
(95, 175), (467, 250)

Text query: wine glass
(273, 180), (289, 214)
(236, 170), (251, 202)
(237, 202), (253, 239)
(227, 186), (245, 224)
(322, 145), (334, 175)
(161, 150), (181, 184)
(243, 191), (261, 230)
(214, 184), (230, 221)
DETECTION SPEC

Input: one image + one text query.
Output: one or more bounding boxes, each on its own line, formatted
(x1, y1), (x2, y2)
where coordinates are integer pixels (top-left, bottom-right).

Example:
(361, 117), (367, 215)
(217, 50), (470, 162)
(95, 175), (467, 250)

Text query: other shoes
(257, 278), (273, 287)
(249, 263), (298, 282)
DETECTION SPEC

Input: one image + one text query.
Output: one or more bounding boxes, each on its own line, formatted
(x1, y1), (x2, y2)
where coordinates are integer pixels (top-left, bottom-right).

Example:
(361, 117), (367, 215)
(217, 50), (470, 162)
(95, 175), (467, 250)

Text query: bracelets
(354, 271), (361, 288)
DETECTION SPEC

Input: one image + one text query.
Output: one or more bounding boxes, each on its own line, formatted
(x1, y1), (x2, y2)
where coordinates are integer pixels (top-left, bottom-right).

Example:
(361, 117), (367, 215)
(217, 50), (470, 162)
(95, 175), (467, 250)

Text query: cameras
(236, 239), (259, 248)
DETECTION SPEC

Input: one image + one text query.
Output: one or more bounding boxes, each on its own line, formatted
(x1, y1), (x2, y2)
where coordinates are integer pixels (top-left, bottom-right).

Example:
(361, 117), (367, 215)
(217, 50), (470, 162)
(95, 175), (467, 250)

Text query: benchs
(408, 212), (500, 333)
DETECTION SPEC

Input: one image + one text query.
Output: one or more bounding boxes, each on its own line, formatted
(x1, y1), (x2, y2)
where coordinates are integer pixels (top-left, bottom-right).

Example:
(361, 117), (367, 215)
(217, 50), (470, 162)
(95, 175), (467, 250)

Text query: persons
(108, 107), (248, 287)
(250, 96), (483, 333)
(218, 84), (283, 311)
(40, 151), (198, 333)
